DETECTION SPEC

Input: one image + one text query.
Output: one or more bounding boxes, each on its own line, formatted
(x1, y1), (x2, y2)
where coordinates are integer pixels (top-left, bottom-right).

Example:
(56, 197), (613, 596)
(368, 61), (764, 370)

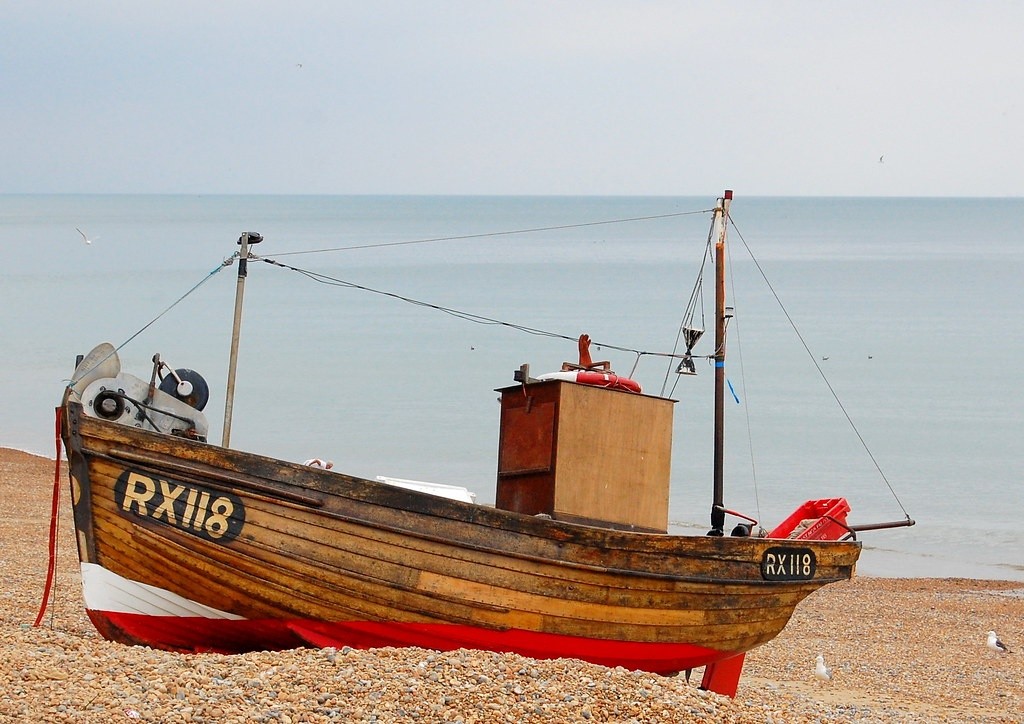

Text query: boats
(59, 189), (918, 701)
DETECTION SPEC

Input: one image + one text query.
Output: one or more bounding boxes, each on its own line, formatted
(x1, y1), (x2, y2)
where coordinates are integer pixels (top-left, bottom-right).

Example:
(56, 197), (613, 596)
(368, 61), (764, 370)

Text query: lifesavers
(576, 373), (641, 393)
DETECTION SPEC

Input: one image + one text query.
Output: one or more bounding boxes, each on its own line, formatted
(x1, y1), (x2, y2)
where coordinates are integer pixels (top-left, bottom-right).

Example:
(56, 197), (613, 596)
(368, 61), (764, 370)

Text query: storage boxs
(763, 498), (852, 542)
